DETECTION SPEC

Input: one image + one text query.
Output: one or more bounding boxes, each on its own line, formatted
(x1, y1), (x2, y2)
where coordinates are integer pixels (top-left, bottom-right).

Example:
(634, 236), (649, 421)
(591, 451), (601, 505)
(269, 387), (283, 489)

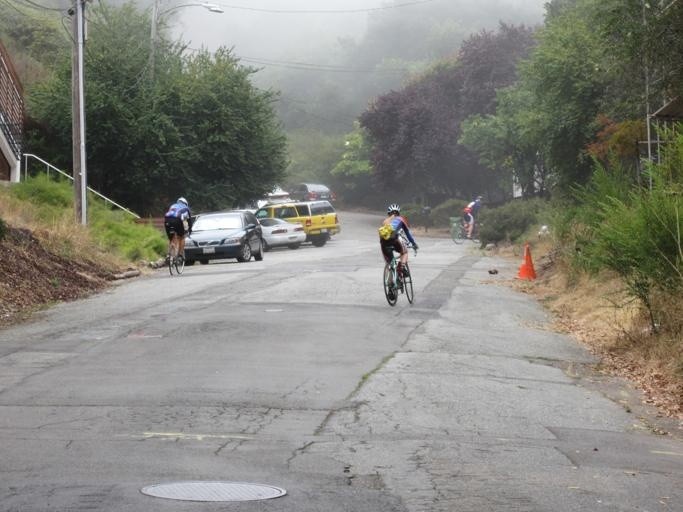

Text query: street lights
(149, 2), (223, 82)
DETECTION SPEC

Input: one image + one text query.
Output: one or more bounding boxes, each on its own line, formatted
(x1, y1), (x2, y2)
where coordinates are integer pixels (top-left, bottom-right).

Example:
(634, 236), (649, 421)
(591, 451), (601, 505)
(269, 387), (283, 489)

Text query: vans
(255, 200), (340, 246)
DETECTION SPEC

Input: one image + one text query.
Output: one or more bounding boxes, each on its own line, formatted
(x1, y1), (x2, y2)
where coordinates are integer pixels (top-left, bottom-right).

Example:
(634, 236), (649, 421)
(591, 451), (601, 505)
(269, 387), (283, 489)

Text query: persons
(378, 204), (417, 294)
(163, 197), (192, 261)
(462, 195), (483, 238)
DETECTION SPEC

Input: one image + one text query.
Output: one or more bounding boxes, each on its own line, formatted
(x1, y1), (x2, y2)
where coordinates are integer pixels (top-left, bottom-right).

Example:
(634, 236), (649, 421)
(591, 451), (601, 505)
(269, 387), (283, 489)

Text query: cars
(257, 184), (336, 209)
(184, 209), (262, 265)
(255, 217), (306, 252)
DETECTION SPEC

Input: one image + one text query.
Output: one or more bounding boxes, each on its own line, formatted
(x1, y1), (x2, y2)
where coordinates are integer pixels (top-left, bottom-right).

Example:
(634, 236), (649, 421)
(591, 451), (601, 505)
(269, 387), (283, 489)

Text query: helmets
(387, 203), (401, 215)
(176, 197), (188, 206)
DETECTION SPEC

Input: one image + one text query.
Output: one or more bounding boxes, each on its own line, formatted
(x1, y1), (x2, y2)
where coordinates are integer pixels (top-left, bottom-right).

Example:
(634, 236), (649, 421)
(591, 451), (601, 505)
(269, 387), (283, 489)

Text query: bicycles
(166, 225), (188, 276)
(382, 245), (416, 306)
(451, 218), (484, 244)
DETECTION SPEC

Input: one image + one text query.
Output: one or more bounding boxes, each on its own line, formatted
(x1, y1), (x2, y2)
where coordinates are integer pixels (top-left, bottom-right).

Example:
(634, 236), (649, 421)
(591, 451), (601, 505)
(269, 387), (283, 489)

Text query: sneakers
(397, 262), (409, 277)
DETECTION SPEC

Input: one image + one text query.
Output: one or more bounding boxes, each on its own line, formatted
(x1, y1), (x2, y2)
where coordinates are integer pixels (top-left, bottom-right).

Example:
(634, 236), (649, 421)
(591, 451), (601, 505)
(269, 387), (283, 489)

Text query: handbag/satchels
(378, 224), (394, 240)
(464, 208), (472, 213)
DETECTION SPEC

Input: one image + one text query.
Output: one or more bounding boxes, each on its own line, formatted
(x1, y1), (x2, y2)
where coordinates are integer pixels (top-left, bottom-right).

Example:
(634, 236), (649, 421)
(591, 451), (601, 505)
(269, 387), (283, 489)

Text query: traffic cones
(518, 246), (535, 281)
